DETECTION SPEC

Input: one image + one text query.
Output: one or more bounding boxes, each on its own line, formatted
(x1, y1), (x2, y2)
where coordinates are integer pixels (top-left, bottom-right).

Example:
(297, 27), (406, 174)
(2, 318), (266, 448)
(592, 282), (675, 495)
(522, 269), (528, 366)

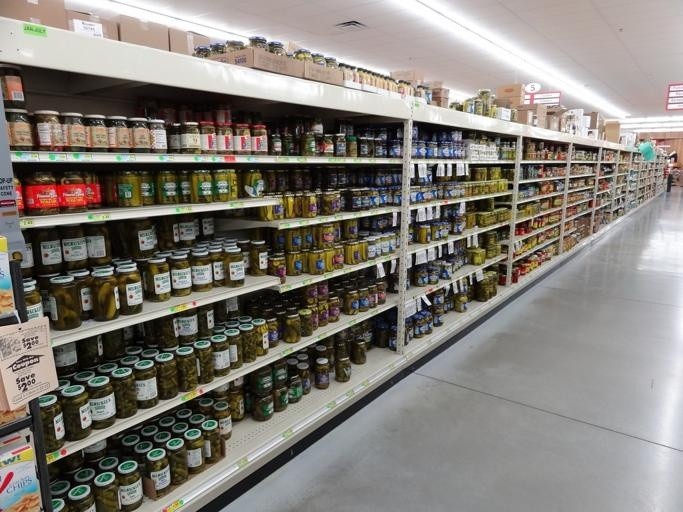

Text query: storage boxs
(584, 112), (598, 129)
(116, 13), (170, 52)
(431, 88), (448, 107)
(169, 26), (211, 59)
(605, 122), (619, 142)
(494, 83), (561, 131)
(207, 51), (305, 78)
(66, 7), (120, 39)
(1, 0), (67, 37)
(304, 61), (345, 87)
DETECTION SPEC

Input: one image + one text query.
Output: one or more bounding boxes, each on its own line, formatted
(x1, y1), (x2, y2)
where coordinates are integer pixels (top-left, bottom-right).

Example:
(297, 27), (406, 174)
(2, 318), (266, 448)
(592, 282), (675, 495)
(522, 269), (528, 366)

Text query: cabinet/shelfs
(404, 101), (519, 360)
(512, 121), (616, 285)
(610, 146), (666, 221)
(0, 26), (404, 512)
